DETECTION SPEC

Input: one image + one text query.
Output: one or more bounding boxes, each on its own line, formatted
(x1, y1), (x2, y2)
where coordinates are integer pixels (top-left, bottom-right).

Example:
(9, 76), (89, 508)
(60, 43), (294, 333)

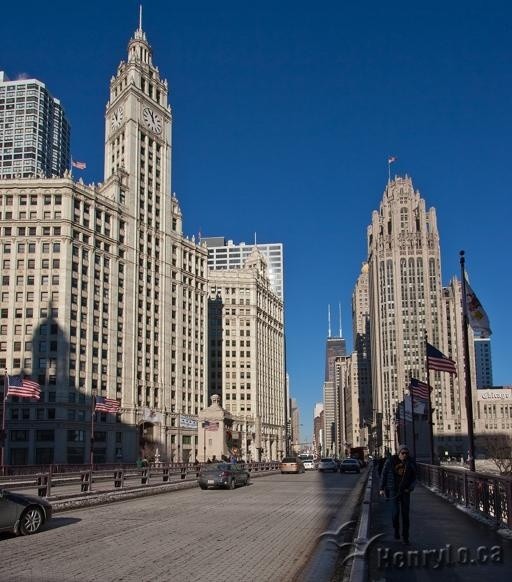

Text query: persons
(141, 456), (149, 476)
(379, 445), (417, 545)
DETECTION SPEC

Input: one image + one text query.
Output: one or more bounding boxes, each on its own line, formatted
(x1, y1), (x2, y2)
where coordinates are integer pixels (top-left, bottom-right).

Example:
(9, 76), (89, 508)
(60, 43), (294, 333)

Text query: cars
(318, 457), (360, 473)
(0, 488), (52, 536)
(197, 462), (250, 490)
(302, 460), (315, 470)
(279, 457), (305, 474)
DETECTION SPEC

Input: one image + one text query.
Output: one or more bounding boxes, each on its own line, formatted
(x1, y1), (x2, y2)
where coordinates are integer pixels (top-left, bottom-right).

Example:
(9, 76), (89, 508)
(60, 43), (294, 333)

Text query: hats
(397, 444), (409, 455)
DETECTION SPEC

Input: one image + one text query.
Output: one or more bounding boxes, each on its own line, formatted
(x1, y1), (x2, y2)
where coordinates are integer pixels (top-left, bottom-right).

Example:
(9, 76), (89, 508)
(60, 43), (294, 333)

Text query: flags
(180, 415), (198, 428)
(7, 376), (41, 400)
(427, 343), (457, 378)
(399, 378), (433, 422)
(463, 279), (493, 338)
(144, 408), (162, 422)
(95, 396), (120, 413)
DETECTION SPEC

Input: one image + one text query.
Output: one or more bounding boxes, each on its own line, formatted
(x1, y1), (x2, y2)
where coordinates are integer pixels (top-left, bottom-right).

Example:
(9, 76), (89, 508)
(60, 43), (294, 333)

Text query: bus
(298, 453), (315, 461)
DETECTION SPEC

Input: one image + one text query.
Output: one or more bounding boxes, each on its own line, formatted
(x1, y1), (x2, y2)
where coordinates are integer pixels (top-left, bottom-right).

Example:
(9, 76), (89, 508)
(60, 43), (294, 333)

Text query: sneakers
(403, 537), (410, 545)
(394, 533), (400, 540)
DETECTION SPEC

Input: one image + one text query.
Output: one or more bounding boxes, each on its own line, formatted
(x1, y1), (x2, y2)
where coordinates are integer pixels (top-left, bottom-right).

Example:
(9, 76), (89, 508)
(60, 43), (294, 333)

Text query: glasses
(400, 451), (408, 455)
